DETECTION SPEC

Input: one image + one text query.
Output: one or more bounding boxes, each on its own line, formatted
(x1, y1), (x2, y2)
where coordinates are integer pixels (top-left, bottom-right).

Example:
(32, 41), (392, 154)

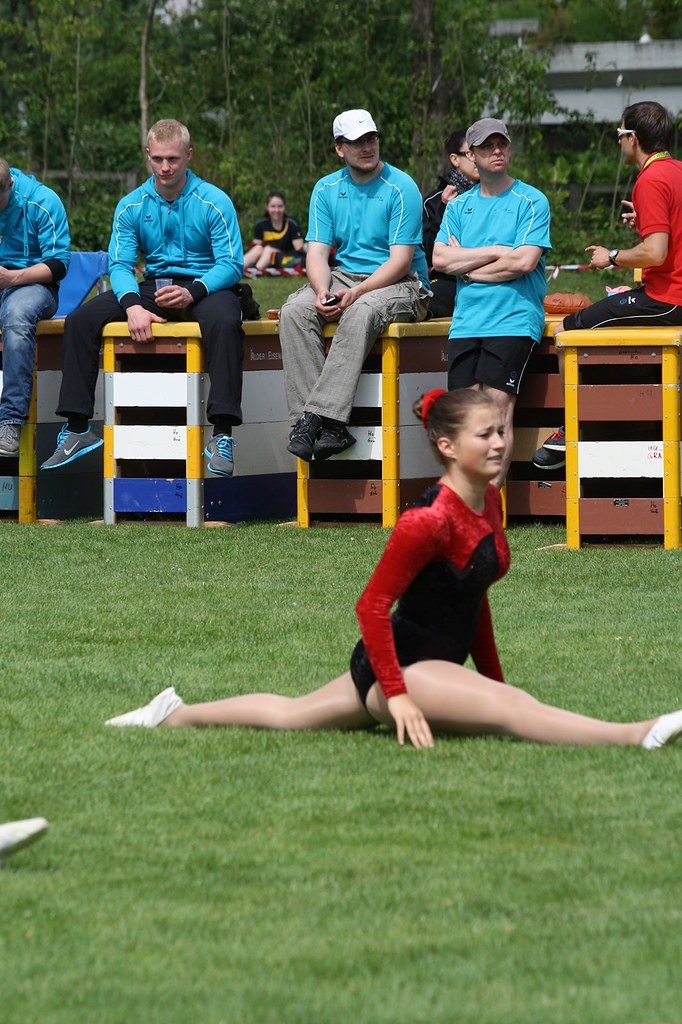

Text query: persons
(0, 815), (49, 860)
(41, 119), (246, 477)
(0, 156), (71, 457)
(531, 101), (682, 470)
(431, 119), (551, 489)
(279, 109), (432, 462)
(422, 128), (481, 318)
(103, 392), (682, 752)
(243, 193), (304, 270)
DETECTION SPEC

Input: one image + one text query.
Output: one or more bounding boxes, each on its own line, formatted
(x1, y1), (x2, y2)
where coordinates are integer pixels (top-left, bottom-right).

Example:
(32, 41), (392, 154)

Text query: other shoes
(0, 816), (49, 860)
(640, 709), (682, 749)
(105, 685), (183, 728)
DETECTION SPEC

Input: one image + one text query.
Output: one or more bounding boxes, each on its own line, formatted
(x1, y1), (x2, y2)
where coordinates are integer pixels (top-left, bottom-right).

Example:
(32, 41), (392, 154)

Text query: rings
(590, 259), (593, 263)
(176, 300), (183, 308)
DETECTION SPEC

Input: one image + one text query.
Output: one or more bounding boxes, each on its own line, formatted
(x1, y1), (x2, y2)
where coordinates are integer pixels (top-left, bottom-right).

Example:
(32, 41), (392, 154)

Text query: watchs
(608, 248), (620, 267)
(460, 273), (472, 284)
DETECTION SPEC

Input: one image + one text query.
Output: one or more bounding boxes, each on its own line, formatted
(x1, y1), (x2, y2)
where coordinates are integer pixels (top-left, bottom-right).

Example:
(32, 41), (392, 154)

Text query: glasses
(616, 127), (636, 139)
(341, 133), (379, 146)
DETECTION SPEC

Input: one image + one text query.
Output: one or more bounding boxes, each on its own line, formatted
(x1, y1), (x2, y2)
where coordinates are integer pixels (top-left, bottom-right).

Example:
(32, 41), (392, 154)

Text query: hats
(466, 118), (512, 149)
(333, 109), (378, 141)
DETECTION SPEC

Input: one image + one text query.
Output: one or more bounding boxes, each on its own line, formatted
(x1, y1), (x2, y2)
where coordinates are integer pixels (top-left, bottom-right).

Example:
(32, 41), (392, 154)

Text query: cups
(155, 278), (174, 302)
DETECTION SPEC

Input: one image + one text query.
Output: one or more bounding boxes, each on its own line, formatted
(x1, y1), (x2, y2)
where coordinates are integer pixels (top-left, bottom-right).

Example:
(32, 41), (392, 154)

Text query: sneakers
(313, 419), (357, 461)
(205, 433), (237, 478)
(531, 425), (569, 470)
(0, 424), (23, 457)
(286, 412), (322, 462)
(41, 422), (104, 472)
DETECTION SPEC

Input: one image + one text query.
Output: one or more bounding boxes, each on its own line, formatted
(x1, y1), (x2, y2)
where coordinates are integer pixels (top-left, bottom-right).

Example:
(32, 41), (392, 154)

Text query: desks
(0, 313), (682, 551)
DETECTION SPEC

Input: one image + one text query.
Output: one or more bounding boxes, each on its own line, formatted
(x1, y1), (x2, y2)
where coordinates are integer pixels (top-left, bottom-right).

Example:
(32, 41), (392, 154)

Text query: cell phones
(324, 296), (340, 306)
